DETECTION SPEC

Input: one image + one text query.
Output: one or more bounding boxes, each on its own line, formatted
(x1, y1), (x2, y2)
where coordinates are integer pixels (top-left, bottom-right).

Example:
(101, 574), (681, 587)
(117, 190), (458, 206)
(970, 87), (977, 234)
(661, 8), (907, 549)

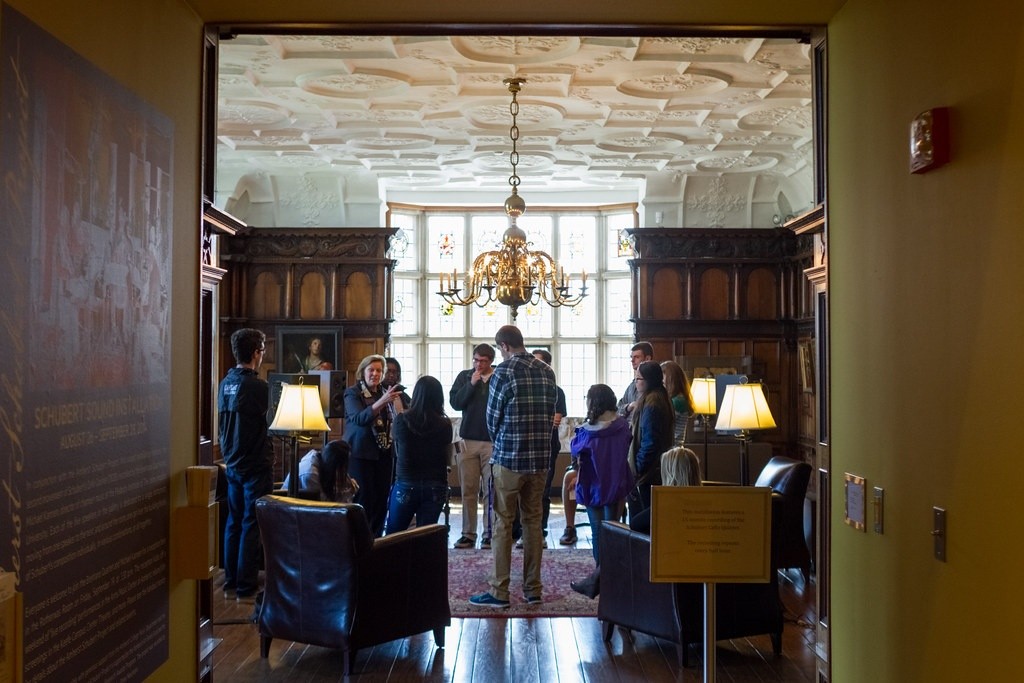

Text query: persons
(660, 359), (695, 445)
(280, 439), (354, 502)
(380, 375), (453, 537)
(447, 342), (498, 550)
(218, 327), (273, 605)
(559, 386), (634, 569)
(515, 348), (568, 548)
(626, 360), (676, 520)
(618, 341), (654, 430)
(468, 325), (557, 608)
(382, 355), (412, 411)
(569, 446), (703, 600)
(340, 353), (404, 540)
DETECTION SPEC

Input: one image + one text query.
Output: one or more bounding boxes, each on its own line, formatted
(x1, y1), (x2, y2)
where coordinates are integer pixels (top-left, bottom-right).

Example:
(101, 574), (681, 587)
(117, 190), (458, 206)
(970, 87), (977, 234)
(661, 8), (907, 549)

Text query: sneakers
(561, 527), (577, 543)
(480, 537), (492, 549)
(454, 536), (475, 547)
(522, 594), (542, 605)
(469, 592), (511, 607)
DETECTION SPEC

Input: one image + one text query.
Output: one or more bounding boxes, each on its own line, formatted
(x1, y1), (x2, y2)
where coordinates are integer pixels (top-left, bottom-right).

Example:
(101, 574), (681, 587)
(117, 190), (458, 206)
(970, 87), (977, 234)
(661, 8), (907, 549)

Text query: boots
(570, 566), (601, 599)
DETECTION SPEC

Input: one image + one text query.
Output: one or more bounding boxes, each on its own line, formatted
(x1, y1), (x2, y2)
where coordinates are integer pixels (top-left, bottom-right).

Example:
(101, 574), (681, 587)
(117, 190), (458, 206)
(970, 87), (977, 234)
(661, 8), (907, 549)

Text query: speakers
(309, 369), (348, 418)
(716, 373), (758, 435)
(268, 372), (322, 438)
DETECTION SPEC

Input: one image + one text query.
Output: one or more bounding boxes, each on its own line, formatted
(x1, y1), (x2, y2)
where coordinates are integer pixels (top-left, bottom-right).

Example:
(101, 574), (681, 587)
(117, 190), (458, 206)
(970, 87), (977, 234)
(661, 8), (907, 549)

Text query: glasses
(258, 347), (267, 355)
(634, 375), (644, 383)
(472, 358), (491, 365)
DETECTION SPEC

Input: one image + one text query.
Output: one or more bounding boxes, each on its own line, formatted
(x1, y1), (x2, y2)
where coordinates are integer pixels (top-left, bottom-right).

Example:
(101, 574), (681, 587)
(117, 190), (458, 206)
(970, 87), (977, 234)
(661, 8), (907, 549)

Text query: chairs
(565, 421), (633, 525)
(756, 453), (813, 586)
(598, 513), (785, 670)
(215, 463), (321, 568)
(254, 493), (451, 676)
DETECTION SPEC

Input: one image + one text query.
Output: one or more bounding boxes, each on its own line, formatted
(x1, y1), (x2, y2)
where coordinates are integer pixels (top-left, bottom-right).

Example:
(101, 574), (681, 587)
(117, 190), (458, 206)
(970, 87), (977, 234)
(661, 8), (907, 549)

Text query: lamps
(269, 377), (331, 496)
(772, 214), (795, 229)
(436, 75), (588, 322)
(690, 375), (717, 479)
(716, 383), (777, 486)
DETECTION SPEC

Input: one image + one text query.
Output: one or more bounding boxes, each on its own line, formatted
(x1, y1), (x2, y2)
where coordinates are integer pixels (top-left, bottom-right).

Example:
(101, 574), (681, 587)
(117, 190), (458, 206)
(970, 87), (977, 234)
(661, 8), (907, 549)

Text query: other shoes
(541, 535), (547, 548)
(236, 587), (265, 603)
(516, 535), (523, 549)
(224, 586), (237, 600)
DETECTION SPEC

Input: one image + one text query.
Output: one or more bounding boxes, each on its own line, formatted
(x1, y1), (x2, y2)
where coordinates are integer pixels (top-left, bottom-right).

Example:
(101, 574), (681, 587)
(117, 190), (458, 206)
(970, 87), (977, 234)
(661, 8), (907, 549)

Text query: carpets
(257, 546), (600, 616)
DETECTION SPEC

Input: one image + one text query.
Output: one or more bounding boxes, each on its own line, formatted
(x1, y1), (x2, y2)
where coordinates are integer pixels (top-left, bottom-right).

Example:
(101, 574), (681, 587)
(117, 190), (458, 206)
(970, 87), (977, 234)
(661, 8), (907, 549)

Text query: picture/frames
(683, 355), (747, 434)
(275, 325), (343, 375)
